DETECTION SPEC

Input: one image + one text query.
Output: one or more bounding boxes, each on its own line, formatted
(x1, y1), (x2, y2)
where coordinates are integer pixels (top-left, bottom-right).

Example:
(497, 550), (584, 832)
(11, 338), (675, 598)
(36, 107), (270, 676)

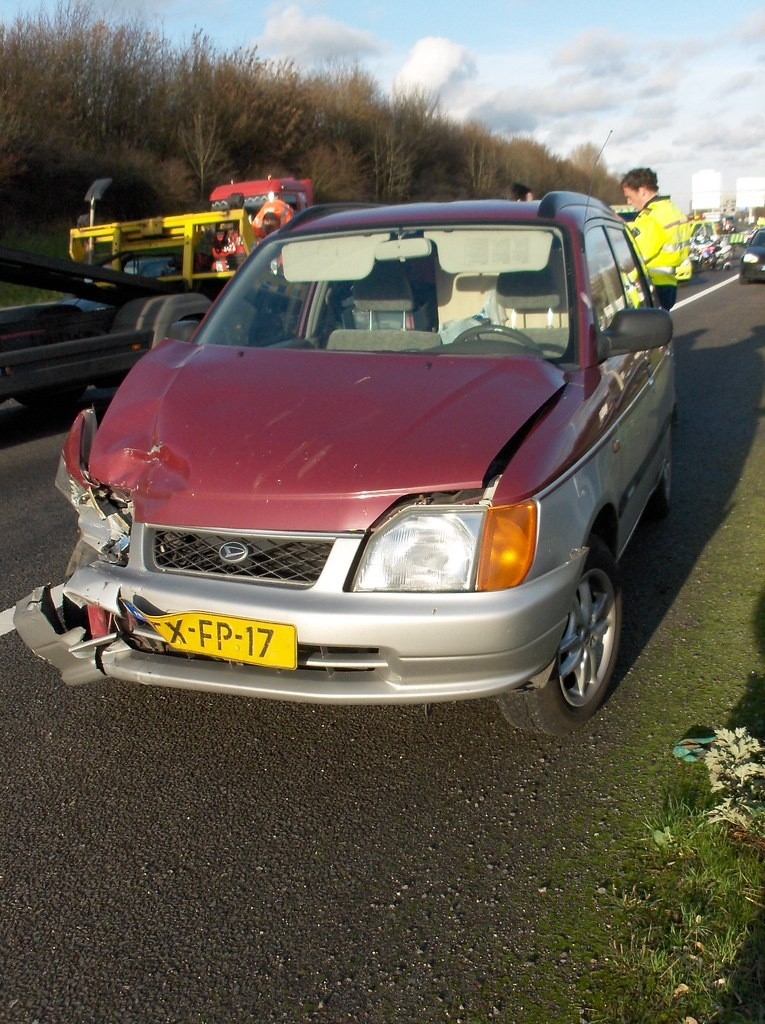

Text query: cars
(739, 226), (765, 285)
(12, 189), (680, 737)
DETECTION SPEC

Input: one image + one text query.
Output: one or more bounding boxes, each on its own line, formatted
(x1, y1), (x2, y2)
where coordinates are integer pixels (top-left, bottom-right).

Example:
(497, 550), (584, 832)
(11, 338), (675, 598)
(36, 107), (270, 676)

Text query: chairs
(479, 265), (569, 358)
(326, 269), (442, 352)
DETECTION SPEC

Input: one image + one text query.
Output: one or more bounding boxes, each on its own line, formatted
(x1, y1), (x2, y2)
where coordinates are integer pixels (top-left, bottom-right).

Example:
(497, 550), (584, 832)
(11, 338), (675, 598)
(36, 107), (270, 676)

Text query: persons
(619, 169), (692, 423)
(508, 184), (534, 203)
(255, 213), (278, 244)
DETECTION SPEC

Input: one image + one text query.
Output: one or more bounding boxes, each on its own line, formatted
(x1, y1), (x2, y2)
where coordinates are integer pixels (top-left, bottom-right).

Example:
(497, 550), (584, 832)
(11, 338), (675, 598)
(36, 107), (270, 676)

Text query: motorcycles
(688, 225), (733, 273)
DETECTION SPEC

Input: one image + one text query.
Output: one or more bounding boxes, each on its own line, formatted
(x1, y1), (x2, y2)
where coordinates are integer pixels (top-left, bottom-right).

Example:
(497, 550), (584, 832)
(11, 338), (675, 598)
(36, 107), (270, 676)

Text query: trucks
(702, 211), (736, 234)
(0, 176), (314, 423)
